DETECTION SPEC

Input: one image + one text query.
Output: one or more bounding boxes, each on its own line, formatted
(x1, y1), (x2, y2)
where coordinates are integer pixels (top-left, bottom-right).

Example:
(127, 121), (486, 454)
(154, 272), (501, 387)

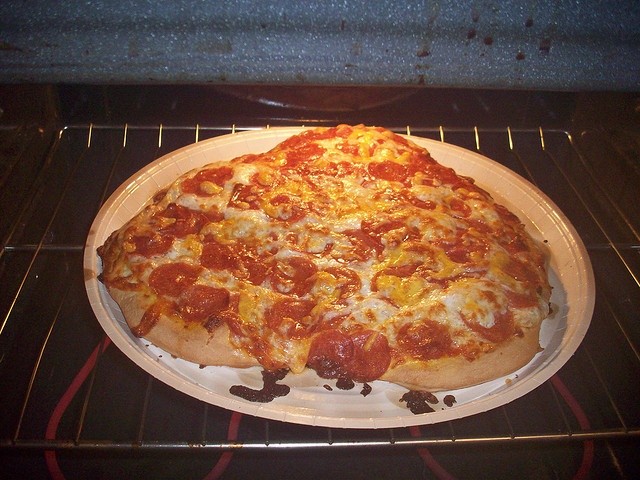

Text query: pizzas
(96, 122), (552, 393)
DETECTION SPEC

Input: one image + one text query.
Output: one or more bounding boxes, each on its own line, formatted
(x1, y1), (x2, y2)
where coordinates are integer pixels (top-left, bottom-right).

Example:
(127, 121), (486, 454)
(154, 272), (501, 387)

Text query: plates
(80, 123), (597, 432)
